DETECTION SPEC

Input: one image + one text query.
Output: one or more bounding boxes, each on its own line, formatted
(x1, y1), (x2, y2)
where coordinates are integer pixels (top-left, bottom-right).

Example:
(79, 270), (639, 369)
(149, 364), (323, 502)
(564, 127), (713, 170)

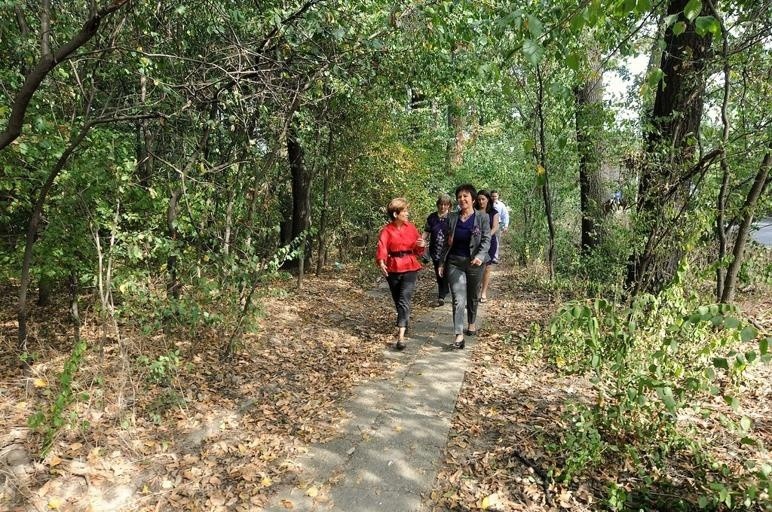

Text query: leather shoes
(467, 322), (476, 336)
(480, 296), (487, 303)
(451, 339), (466, 349)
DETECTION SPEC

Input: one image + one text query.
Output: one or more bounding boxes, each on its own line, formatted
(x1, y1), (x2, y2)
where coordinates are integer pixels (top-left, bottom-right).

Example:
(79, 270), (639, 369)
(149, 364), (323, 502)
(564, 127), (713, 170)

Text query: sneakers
(438, 298), (444, 306)
(396, 341), (406, 350)
(492, 260), (498, 264)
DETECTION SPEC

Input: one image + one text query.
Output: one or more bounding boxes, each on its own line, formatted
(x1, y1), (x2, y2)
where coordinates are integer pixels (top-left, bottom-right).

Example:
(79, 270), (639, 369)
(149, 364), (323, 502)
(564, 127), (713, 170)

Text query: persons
(376, 197), (428, 350)
(422, 189), (508, 308)
(434, 182), (493, 350)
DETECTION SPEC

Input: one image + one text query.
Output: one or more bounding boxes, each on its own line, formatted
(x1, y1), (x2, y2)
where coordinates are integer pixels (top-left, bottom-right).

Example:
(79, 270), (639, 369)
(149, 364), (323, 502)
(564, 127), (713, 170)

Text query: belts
(389, 249), (414, 257)
